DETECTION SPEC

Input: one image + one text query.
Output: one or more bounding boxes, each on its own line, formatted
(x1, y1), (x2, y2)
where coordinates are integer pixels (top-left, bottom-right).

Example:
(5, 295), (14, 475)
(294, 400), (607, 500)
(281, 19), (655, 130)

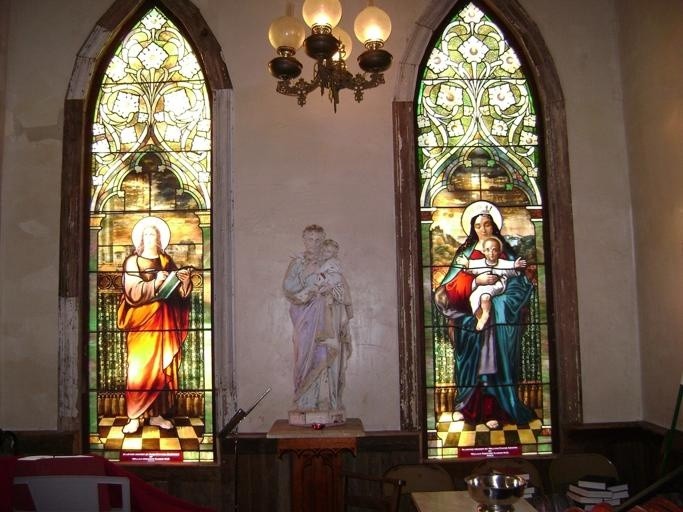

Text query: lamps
(264, 1), (395, 114)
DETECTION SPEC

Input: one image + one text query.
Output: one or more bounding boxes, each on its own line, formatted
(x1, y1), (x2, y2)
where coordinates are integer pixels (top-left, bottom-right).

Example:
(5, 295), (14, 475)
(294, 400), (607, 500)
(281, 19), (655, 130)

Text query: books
(159, 271), (183, 299)
(567, 477), (629, 510)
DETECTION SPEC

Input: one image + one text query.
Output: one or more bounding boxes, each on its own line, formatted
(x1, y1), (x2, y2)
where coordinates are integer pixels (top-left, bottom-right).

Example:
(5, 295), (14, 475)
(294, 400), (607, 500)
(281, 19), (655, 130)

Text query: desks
(408, 483), (540, 512)
(262, 417), (368, 511)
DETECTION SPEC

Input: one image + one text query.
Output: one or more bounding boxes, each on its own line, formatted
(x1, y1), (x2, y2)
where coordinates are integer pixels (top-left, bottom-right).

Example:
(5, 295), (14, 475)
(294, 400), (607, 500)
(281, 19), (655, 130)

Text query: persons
(315, 239), (340, 300)
(282, 225), (352, 412)
(456, 237), (527, 330)
(118, 218), (193, 434)
(435, 202), (535, 429)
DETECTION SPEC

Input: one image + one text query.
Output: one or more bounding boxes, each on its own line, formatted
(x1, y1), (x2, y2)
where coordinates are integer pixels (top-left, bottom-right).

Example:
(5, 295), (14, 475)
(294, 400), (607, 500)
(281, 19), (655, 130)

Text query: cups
(311, 423), (322, 430)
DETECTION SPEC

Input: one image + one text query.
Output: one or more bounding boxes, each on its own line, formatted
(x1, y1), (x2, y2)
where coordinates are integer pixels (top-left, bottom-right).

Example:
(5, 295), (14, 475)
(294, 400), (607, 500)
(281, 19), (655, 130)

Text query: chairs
(545, 451), (648, 511)
(337, 466), (406, 511)
(10, 471), (131, 511)
(380, 462), (458, 511)
(461, 456), (553, 512)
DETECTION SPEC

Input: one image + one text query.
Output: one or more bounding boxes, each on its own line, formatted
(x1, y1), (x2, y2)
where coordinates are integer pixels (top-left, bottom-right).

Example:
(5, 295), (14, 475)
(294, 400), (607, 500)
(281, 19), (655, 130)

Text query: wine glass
(464, 471), (526, 511)
